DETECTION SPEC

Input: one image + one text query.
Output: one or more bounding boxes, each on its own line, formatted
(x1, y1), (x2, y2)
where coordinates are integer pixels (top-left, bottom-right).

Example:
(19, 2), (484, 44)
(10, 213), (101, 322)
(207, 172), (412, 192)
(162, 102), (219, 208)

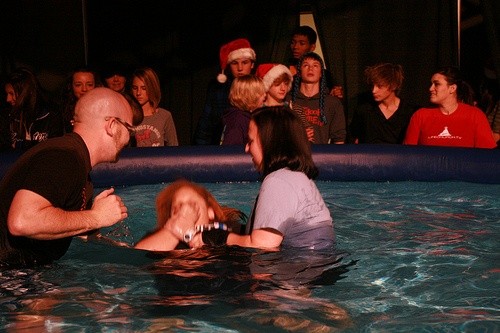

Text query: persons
(134, 178), (249, 252)
(197, 25), (500, 148)
(0, 87), (128, 271)
(0, 64), (143, 157)
(188, 107), (334, 253)
(131, 67), (178, 147)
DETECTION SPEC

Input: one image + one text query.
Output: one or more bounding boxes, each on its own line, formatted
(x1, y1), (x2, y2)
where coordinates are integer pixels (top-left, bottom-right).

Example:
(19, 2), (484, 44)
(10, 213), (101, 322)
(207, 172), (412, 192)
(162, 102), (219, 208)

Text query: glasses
(105, 115), (136, 137)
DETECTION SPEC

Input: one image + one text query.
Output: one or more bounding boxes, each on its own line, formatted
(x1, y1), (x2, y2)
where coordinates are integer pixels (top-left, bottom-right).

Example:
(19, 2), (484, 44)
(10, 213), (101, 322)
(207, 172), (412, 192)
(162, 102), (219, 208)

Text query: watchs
(184, 228), (197, 242)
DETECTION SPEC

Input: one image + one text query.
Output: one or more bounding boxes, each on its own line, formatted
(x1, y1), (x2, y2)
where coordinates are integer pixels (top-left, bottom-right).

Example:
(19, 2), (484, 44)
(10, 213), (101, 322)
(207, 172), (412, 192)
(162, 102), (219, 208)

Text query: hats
(217, 38), (256, 83)
(255, 62), (294, 92)
